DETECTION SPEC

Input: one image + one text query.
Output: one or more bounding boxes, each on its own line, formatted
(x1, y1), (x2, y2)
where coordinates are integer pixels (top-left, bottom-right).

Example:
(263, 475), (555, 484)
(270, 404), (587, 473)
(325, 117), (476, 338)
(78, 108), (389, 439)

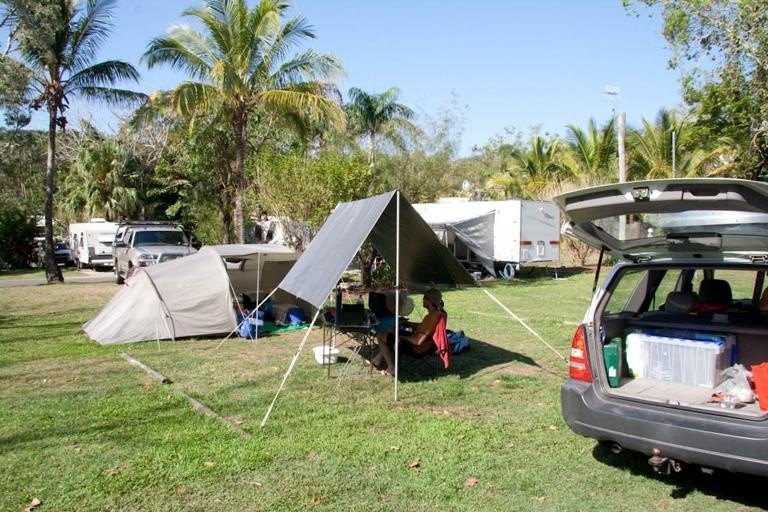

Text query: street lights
(604, 85), (627, 241)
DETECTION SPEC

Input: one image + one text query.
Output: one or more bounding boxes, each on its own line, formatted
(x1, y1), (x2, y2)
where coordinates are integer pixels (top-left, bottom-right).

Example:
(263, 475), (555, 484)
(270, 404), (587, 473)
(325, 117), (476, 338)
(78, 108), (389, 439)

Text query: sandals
(381, 368), (395, 376)
(370, 358), (381, 366)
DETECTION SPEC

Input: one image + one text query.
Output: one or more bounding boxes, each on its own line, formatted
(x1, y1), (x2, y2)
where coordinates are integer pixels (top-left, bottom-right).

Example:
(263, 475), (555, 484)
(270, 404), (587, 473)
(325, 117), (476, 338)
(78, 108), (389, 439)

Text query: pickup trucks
(112, 221), (199, 284)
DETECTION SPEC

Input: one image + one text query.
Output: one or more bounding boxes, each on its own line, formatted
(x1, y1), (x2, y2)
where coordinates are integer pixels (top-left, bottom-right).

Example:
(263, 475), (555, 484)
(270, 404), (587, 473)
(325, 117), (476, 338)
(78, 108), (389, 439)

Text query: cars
(37, 237), (70, 267)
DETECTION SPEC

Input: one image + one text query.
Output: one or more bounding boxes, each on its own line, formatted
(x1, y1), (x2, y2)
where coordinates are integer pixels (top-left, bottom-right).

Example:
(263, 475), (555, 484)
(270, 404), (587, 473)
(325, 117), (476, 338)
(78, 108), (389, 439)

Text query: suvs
(552, 177), (767, 476)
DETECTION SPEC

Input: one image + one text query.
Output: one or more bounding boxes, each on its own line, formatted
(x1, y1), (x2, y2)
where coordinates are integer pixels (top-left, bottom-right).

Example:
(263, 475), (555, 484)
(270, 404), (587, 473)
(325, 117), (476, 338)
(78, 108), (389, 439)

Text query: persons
(371, 286), (445, 377)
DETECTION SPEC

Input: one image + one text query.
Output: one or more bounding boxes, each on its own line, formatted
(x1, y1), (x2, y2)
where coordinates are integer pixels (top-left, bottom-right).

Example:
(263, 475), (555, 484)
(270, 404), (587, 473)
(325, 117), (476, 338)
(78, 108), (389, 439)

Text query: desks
(320, 311), (378, 377)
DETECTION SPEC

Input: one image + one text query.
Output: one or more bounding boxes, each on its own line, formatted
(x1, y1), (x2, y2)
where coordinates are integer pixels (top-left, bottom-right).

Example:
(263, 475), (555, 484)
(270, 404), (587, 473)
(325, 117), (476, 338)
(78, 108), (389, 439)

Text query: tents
(81, 242), (329, 346)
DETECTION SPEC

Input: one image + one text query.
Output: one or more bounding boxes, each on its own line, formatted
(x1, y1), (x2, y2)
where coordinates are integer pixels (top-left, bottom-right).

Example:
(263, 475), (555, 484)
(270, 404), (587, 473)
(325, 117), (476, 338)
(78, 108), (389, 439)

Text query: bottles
(356, 294), (364, 305)
